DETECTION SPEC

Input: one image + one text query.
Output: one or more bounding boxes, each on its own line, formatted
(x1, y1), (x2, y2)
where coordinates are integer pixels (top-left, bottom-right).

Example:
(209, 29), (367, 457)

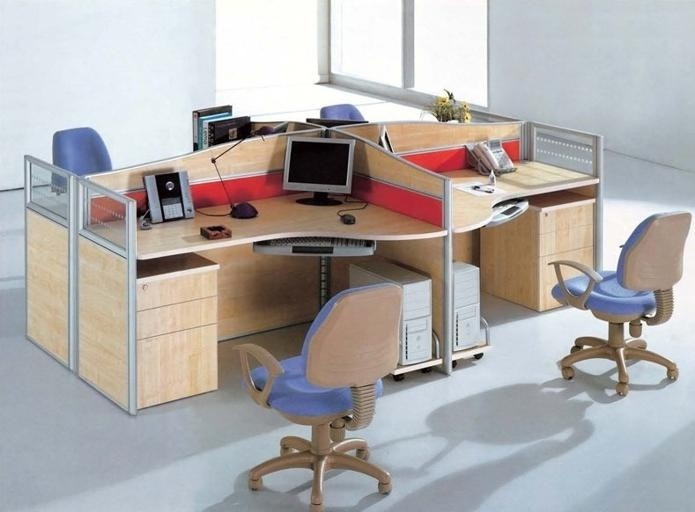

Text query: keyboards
(489, 198), (529, 223)
(253, 238), (374, 256)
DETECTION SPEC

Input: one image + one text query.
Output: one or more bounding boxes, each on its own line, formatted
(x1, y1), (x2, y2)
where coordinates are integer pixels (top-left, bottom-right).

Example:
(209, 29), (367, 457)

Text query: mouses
(340, 214), (355, 224)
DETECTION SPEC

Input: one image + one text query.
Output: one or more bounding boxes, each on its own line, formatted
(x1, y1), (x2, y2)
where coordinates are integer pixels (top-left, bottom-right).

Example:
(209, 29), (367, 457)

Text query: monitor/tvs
(272, 122), (288, 134)
(306, 118), (369, 128)
(283, 135), (356, 206)
(379, 125), (394, 153)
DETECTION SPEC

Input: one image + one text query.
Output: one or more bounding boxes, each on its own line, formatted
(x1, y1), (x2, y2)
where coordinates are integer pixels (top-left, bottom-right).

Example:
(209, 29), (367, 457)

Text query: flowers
(425, 91), (472, 123)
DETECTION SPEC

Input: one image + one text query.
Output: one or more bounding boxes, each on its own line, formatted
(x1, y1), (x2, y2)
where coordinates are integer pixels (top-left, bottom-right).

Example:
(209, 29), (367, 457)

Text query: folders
(192, 105), (251, 152)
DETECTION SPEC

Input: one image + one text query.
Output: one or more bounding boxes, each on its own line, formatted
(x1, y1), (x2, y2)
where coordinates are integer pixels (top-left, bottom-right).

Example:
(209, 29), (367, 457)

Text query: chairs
(548, 209), (694, 401)
(321, 103), (364, 121)
(233, 282), (405, 512)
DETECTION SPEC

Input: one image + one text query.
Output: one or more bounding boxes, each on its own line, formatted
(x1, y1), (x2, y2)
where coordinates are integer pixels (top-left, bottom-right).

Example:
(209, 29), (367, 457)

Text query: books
(192, 105), (251, 154)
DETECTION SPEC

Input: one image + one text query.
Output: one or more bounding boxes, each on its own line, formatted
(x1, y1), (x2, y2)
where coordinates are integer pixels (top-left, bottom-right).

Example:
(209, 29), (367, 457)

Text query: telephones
(473, 138), (518, 173)
(143, 169), (195, 224)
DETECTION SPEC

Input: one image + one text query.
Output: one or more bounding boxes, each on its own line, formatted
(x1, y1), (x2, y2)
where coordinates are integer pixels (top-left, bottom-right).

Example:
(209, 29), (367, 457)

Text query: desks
(22, 109), (605, 416)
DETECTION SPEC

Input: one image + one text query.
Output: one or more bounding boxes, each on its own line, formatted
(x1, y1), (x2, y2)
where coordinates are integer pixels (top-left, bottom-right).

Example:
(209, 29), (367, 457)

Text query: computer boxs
(349, 257), (432, 365)
(453, 261), (481, 351)
